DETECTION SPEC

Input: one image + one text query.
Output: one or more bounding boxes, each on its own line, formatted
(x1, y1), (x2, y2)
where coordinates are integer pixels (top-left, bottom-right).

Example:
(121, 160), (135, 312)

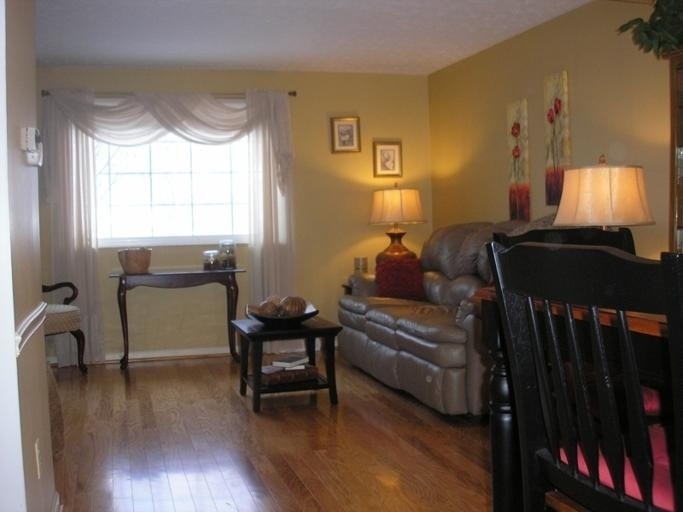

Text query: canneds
(203, 240), (236, 270)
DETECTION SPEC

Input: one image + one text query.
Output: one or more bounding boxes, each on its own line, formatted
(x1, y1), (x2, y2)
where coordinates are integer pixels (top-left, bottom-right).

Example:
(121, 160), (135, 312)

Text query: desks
(108, 268), (247, 368)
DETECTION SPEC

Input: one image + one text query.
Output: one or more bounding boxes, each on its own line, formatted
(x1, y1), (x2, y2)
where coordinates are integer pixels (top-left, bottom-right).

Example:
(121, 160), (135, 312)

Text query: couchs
(339, 218), (529, 422)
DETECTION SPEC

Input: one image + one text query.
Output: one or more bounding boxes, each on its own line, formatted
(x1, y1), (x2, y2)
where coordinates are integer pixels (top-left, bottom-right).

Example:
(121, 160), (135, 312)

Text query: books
(283, 359), (309, 372)
(271, 356), (310, 369)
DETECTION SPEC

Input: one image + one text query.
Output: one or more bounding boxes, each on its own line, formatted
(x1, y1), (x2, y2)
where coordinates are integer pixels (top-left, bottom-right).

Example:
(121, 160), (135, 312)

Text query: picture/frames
(372, 140), (403, 177)
(331, 116), (361, 152)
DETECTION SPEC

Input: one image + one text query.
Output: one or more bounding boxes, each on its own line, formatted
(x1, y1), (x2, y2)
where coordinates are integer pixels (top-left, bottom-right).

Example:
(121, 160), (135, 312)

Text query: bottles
(353, 256), (368, 275)
(201, 250), (219, 270)
(218, 239), (236, 270)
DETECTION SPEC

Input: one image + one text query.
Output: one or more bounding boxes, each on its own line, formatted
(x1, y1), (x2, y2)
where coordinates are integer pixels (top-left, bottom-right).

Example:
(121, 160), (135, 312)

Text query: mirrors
(661, 49), (683, 255)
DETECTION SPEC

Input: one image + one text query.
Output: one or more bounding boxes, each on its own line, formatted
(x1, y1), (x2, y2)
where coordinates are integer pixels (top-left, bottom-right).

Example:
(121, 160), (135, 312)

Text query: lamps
(370, 182), (428, 261)
(552, 156), (656, 228)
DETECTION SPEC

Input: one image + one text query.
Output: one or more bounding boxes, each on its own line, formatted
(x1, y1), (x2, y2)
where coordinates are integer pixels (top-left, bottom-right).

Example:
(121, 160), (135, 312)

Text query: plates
(244, 301), (319, 327)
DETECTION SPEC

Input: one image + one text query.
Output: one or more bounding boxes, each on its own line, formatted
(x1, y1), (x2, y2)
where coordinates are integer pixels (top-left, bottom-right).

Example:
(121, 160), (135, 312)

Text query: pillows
(377, 254), (426, 299)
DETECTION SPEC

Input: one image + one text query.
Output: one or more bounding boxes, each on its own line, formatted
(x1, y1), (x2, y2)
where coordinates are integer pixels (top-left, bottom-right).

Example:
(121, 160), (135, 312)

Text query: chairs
(484, 243), (683, 512)
(493, 229), (639, 259)
(42, 281), (92, 374)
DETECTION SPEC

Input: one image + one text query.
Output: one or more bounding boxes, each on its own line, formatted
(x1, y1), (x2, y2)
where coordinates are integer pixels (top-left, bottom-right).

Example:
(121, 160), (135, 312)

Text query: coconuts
(257, 295), (307, 315)
